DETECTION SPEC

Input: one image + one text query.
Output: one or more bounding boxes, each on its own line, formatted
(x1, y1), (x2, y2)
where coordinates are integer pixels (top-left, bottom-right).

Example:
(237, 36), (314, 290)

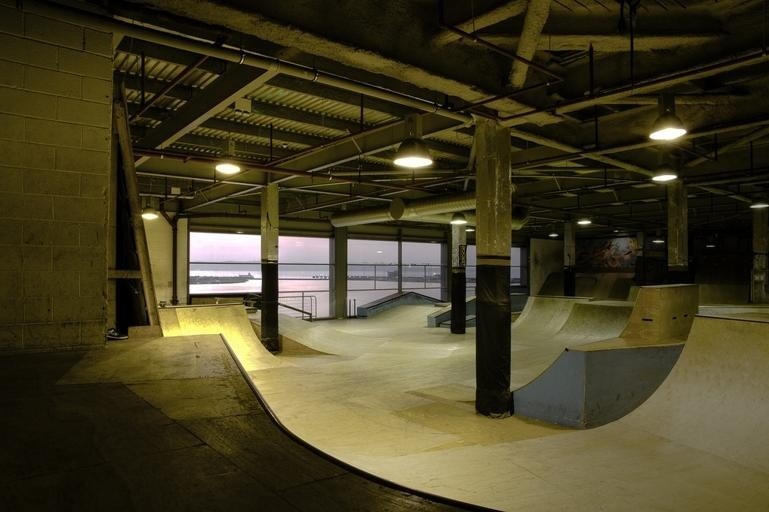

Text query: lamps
(649, 93), (688, 141)
(393, 131), (433, 168)
(216, 164), (240, 176)
(142, 208), (159, 220)
(748, 200), (769, 208)
(651, 166), (678, 182)
